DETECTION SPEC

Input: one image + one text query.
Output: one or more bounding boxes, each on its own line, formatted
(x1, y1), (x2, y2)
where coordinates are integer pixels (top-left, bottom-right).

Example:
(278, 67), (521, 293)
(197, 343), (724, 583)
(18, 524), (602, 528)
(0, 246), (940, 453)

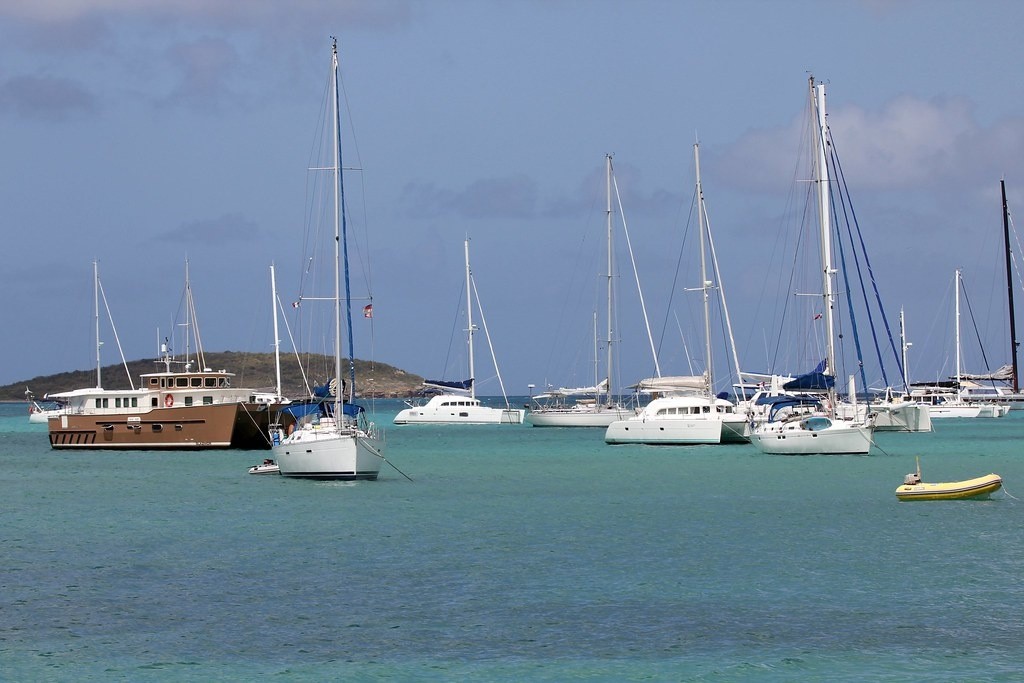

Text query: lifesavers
(165, 394), (174, 408)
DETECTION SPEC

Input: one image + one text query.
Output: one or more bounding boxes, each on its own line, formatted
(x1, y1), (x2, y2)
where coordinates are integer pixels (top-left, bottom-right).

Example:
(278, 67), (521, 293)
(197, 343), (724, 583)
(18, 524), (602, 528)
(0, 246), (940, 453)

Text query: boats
(249, 463), (279, 474)
(895, 455), (1003, 501)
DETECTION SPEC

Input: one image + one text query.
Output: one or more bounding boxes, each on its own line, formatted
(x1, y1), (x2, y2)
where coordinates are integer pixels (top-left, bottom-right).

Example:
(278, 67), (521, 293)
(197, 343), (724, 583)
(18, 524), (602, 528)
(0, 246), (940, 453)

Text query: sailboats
(271, 35), (384, 480)
(26, 253), (313, 450)
(527, 71), (1023, 456)
(392, 229), (525, 425)
